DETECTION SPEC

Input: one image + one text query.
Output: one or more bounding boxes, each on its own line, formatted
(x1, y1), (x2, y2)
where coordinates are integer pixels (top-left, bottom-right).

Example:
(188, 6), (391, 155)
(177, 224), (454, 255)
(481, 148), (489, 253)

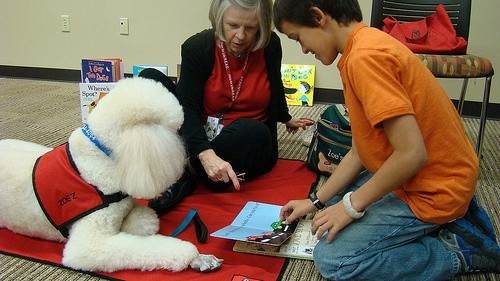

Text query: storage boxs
(79, 82), (120, 123)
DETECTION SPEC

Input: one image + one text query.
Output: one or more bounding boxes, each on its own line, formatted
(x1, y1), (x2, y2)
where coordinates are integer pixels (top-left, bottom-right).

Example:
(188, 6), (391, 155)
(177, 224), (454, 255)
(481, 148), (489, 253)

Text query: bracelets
(309, 190), (326, 210)
(343, 191), (365, 219)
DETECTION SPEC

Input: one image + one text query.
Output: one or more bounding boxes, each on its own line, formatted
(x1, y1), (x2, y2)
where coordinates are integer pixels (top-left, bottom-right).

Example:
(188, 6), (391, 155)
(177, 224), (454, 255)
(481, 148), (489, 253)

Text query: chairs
(370, 0), (494, 162)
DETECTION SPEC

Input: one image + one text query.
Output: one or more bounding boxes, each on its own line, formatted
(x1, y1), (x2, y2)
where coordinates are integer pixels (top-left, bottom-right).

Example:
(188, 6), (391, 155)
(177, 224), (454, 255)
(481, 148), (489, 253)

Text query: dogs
(0, 76), (224, 274)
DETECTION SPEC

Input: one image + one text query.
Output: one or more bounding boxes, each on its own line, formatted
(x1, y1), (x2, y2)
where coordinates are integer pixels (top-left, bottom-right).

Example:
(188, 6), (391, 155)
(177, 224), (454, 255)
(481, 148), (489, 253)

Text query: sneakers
(438, 218), (500, 274)
(463, 194), (498, 244)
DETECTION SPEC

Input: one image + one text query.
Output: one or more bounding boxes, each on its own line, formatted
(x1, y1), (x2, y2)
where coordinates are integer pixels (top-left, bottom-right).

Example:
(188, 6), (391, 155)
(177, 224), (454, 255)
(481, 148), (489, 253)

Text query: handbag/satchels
(381, 3), (468, 55)
(305, 103), (352, 178)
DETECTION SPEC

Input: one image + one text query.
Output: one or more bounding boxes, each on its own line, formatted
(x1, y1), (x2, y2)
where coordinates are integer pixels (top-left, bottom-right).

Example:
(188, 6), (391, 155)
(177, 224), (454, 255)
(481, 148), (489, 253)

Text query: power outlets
(61, 15), (70, 32)
(120, 18), (129, 35)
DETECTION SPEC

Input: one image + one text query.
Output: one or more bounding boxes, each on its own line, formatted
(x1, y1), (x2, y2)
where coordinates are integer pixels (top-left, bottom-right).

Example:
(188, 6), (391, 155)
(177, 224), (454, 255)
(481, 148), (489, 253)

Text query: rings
(217, 177), (224, 182)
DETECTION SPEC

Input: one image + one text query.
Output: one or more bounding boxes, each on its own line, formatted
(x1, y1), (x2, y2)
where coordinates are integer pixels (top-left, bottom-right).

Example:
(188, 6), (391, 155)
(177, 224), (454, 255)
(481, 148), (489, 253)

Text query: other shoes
(147, 166), (197, 216)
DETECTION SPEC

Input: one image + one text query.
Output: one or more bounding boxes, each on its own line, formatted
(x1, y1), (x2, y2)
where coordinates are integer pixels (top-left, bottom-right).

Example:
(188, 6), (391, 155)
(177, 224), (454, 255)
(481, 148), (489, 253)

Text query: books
(79, 58), (168, 122)
(280, 64), (315, 107)
(210, 201), (328, 260)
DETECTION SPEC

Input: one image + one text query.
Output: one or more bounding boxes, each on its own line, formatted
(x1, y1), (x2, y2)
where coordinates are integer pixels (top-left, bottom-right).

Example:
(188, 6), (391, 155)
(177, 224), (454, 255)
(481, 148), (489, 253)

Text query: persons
(138, 0), (317, 215)
(272, 0), (500, 281)
